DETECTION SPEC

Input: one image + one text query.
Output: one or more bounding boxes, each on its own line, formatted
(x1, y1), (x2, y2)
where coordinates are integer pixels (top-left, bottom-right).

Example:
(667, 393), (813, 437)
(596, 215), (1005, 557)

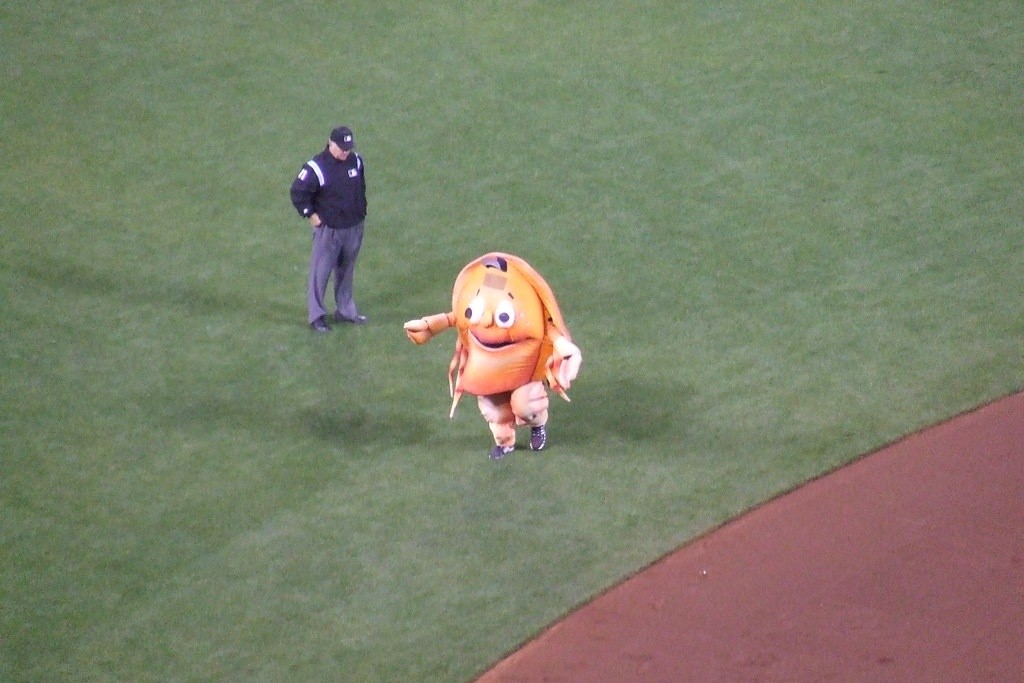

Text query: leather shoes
(334, 310), (367, 324)
(311, 316), (332, 333)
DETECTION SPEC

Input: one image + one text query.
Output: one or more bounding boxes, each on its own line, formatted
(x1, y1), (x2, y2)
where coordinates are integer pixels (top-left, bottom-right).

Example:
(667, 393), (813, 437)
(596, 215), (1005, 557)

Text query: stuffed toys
(403, 252), (582, 459)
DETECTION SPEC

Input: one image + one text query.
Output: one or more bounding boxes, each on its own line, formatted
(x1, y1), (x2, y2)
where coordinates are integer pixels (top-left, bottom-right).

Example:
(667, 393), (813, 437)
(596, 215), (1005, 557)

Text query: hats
(330, 125), (355, 150)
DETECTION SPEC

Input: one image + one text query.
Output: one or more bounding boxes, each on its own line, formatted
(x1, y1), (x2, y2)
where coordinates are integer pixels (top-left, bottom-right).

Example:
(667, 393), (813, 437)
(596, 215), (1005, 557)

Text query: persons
(290, 126), (368, 332)
